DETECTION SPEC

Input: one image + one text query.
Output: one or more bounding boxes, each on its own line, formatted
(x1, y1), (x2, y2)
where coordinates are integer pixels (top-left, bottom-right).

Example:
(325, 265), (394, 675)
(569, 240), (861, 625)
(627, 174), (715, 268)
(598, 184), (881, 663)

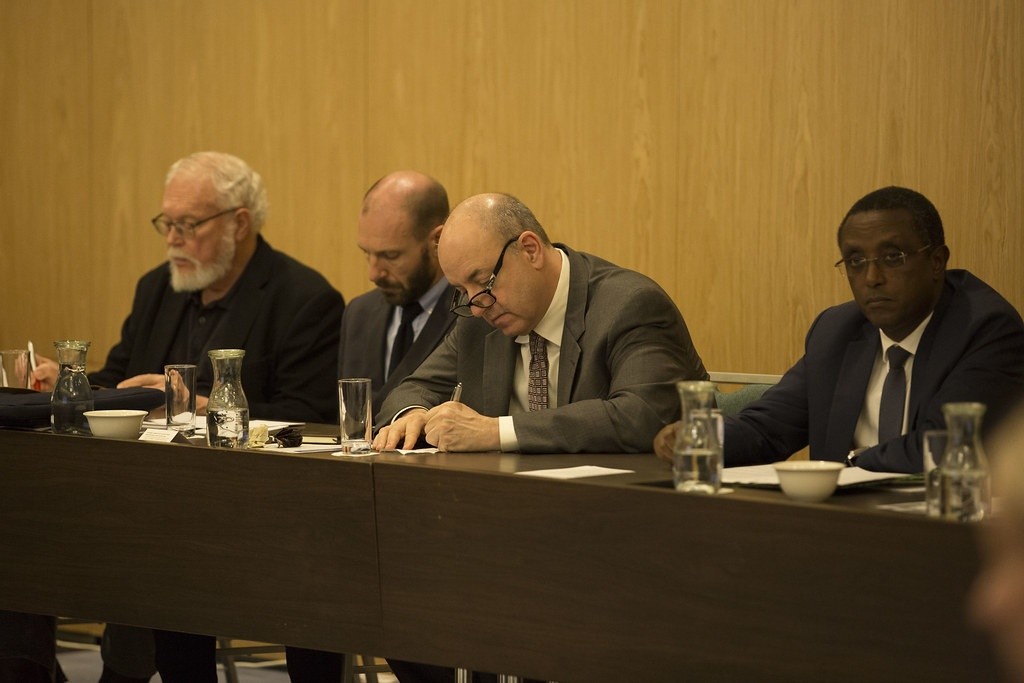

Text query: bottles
(205, 349), (249, 450)
(673, 381), (719, 493)
(942, 404), (992, 522)
(51, 340), (94, 435)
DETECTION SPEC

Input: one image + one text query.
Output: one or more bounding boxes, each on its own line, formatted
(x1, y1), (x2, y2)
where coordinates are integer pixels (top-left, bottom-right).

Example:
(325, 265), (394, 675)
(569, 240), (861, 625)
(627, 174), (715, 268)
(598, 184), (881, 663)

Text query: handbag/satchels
(0, 386), (167, 427)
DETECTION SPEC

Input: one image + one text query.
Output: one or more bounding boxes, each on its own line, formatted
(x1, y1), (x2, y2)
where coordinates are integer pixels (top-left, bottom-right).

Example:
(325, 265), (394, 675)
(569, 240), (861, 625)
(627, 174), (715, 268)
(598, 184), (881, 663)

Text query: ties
(387, 300), (425, 381)
(878, 345), (912, 446)
(528, 330), (549, 418)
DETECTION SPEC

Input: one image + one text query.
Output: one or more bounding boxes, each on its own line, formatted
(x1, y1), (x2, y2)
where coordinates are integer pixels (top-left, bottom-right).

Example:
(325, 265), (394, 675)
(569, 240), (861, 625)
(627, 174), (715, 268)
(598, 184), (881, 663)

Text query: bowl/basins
(83, 411), (148, 440)
(774, 460), (845, 501)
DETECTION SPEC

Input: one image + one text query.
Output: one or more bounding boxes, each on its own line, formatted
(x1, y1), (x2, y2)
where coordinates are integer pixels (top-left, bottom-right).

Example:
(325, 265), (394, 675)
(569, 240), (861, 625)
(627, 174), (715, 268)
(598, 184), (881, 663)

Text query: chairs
(708, 368), (784, 417)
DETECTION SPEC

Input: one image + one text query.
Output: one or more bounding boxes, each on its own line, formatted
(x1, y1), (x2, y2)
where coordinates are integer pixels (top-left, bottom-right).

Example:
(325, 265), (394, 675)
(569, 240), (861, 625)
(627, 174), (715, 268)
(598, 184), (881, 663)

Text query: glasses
(835, 244), (933, 277)
(449, 235), (523, 318)
(151, 206), (251, 240)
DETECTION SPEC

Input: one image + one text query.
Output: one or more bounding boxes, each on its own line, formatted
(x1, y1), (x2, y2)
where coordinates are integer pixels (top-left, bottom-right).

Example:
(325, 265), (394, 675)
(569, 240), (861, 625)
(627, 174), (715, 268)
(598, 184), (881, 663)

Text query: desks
(1, 408), (1024, 682)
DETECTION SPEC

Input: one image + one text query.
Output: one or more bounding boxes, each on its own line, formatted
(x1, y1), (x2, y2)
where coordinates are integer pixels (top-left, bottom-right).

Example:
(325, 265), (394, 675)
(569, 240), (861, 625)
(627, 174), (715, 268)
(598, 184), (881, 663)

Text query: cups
(690, 408), (724, 484)
(164, 364), (196, 439)
(1, 350), (30, 389)
(338, 378), (372, 454)
(925, 431), (951, 517)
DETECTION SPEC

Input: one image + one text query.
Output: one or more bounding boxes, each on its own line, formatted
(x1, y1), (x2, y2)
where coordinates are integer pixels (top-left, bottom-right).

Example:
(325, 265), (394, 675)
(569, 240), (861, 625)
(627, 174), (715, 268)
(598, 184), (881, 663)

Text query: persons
(15, 153), (342, 683)
(371, 191), (717, 453)
(285, 169), (460, 683)
(654, 186), (1024, 473)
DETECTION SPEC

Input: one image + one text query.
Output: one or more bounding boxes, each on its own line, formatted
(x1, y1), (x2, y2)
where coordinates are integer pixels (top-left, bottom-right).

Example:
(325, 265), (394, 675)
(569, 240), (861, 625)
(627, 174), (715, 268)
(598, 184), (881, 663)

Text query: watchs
(847, 446), (868, 467)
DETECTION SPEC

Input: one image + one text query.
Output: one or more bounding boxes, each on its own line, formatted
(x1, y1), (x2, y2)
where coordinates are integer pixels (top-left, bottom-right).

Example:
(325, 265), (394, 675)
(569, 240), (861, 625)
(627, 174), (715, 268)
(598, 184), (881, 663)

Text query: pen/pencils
(27, 340), (41, 391)
(450, 383), (462, 402)
